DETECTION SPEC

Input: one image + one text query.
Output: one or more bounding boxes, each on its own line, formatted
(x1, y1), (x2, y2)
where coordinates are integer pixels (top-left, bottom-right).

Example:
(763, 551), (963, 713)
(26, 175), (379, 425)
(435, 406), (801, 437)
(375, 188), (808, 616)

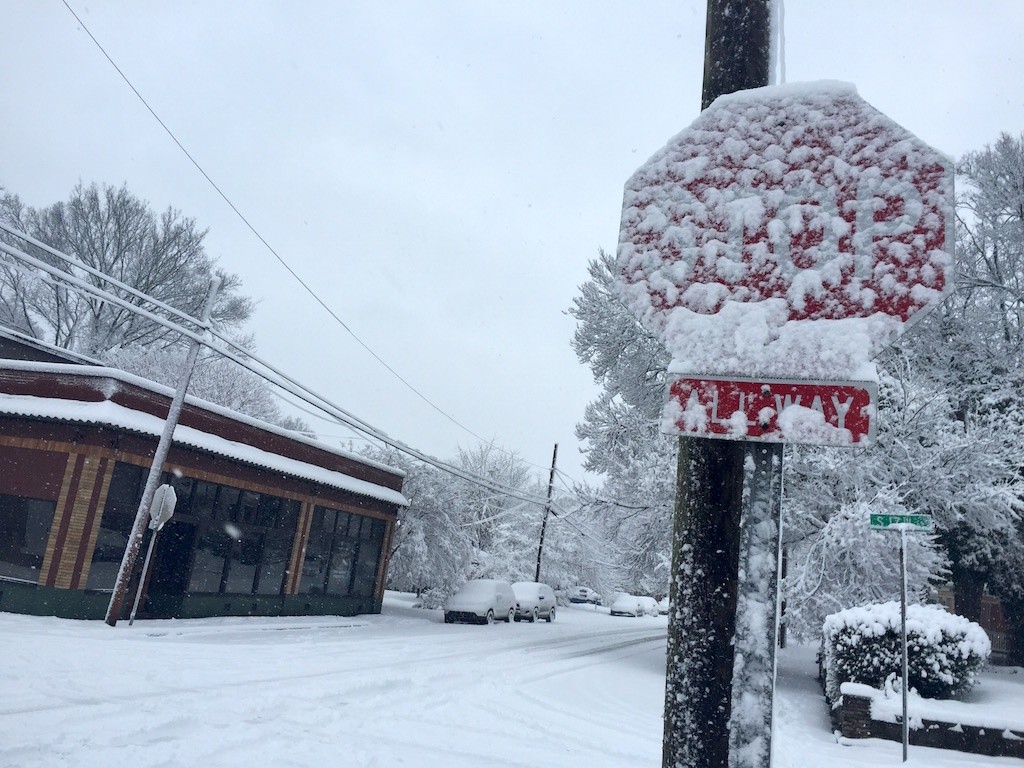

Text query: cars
(609, 596), (670, 618)
(443, 579), (517, 625)
(511, 581), (557, 623)
(566, 585), (602, 604)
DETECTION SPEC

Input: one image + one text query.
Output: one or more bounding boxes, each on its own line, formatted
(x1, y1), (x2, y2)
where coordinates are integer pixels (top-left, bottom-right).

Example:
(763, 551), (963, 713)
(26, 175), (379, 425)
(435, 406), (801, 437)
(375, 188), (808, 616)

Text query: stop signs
(614, 81), (954, 378)
